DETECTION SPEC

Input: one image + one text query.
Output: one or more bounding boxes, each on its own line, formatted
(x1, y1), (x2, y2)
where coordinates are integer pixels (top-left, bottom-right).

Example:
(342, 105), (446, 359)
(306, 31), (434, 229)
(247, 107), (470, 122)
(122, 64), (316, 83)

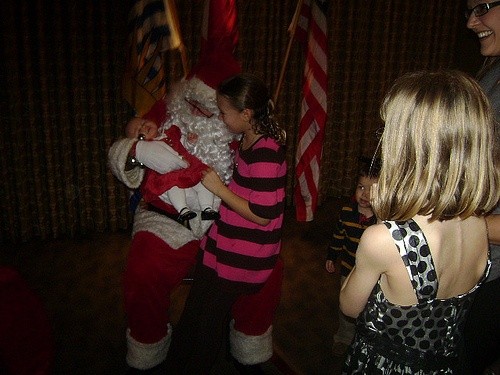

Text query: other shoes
(128, 360), (172, 375)
(233, 356), (281, 375)
(331, 342), (349, 357)
(201, 207), (221, 221)
(179, 208), (197, 221)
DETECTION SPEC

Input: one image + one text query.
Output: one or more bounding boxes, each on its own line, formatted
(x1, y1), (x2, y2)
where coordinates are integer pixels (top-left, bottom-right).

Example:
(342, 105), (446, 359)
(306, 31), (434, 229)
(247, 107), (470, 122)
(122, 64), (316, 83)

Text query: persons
(324, 164), (380, 357)
(106, 49), (284, 375)
(200, 73), (287, 375)
(125, 116), (222, 221)
(339, 70), (500, 375)
(466, 1), (500, 374)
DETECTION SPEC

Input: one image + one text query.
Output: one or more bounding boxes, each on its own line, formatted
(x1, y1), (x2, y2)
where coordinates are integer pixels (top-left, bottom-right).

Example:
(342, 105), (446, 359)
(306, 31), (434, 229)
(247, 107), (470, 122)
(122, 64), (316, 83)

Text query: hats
(180, 47), (243, 102)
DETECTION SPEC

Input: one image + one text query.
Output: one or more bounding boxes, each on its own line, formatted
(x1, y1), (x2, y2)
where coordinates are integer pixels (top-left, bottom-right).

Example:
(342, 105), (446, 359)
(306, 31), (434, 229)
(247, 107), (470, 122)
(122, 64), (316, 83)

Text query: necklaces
(245, 135), (257, 149)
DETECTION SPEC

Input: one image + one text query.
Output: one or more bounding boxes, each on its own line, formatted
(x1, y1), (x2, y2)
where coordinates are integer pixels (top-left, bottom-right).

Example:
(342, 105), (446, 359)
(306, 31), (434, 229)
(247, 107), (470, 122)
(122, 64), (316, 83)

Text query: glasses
(465, 1), (500, 20)
(375, 126), (384, 141)
(184, 97), (214, 119)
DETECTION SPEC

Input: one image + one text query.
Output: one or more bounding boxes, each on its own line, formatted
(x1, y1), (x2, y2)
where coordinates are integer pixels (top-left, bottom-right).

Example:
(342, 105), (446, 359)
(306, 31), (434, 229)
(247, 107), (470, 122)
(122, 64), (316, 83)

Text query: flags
(117, 0), (192, 118)
(205, 0), (244, 90)
(294, 0), (327, 221)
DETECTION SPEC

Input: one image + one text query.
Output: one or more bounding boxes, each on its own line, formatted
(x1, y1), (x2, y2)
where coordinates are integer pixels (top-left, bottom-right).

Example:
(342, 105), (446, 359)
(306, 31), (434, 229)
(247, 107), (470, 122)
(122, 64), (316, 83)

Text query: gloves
(135, 140), (190, 174)
